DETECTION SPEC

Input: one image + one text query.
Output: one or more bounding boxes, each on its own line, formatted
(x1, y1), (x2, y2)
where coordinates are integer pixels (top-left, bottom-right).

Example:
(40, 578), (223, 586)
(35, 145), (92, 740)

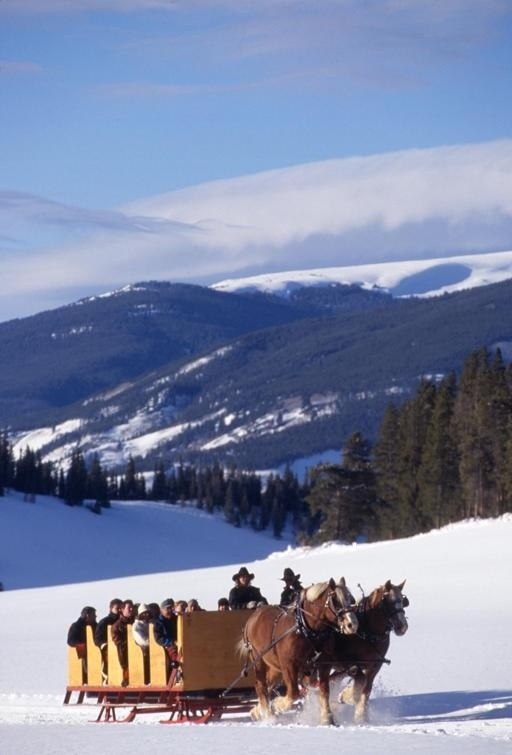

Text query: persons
(280, 567), (304, 608)
(69, 597), (232, 686)
(228, 565), (269, 610)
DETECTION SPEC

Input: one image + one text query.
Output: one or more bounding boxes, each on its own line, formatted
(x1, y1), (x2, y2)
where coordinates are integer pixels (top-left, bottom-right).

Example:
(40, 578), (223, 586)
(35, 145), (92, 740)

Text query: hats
(280, 567), (299, 583)
(233, 567), (255, 581)
(144, 604), (159, 610)
(161, 598), (175, 609)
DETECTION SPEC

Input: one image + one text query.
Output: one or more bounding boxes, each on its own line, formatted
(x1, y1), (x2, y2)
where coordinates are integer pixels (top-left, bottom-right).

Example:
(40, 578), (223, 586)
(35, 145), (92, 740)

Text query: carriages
(65, 575), (410, 728)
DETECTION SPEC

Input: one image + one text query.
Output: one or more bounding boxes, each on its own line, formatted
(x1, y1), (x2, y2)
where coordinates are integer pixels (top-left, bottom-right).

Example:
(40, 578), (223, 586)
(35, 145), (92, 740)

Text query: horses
(300, 578), (409, 722)
(234, 577), (359, 725)
